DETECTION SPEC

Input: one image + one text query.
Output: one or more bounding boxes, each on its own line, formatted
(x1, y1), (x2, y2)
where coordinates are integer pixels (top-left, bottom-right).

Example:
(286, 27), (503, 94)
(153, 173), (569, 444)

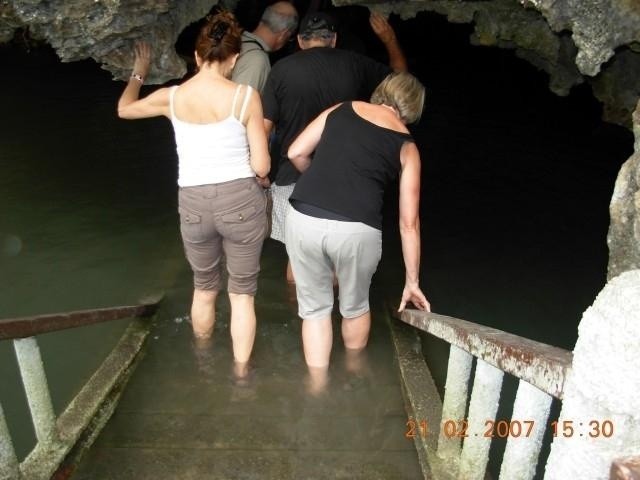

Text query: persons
(229, 0), (300, 90)
(259, 12), (409, 283)
(278, 69), (431, 389)
(116, 9), (270, 385)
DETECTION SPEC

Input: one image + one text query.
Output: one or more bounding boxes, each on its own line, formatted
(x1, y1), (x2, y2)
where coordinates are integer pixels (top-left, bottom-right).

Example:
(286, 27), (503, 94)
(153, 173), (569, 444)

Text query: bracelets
(128, 73), (145, 84)
